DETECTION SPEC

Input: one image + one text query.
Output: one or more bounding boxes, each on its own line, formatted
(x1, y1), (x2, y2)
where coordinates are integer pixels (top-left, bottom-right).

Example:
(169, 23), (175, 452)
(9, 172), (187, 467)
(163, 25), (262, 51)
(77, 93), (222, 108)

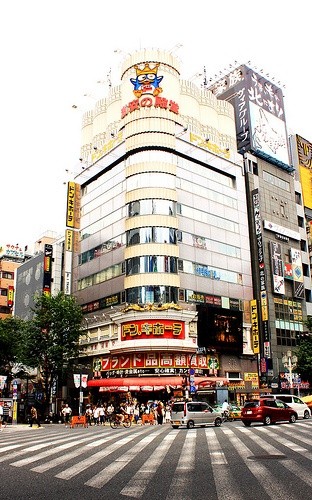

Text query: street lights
(282, 348), (298, 394)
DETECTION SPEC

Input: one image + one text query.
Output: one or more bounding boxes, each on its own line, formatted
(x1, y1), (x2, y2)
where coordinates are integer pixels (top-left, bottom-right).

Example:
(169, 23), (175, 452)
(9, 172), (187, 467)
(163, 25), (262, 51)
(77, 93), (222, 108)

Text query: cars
(299, 395), (312, 410)
(241, 397), (299, 427)
(212, 404), (242, 420)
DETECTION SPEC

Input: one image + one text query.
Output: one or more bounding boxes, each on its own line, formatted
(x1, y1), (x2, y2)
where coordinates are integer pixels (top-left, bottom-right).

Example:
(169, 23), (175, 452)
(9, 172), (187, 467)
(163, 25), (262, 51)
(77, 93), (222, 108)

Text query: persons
(29, 406), (41, 427)
(62, 399), (232, 428)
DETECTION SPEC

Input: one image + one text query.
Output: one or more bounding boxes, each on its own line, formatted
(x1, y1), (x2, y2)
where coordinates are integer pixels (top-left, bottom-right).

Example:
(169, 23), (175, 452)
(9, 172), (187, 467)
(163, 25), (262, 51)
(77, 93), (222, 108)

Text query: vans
(260, 394), (311, 419)
(170, 401), (224, 429)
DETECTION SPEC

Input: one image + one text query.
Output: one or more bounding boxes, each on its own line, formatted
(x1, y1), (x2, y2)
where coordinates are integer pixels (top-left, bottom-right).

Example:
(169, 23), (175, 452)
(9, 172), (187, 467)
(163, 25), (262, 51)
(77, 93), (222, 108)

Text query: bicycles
(110, 412), (132, 429)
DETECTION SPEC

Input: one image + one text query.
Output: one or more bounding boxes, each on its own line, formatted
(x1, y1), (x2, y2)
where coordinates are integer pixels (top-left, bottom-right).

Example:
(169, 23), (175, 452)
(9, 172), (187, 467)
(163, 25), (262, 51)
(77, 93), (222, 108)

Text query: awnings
(86, 377), (229, 391)
